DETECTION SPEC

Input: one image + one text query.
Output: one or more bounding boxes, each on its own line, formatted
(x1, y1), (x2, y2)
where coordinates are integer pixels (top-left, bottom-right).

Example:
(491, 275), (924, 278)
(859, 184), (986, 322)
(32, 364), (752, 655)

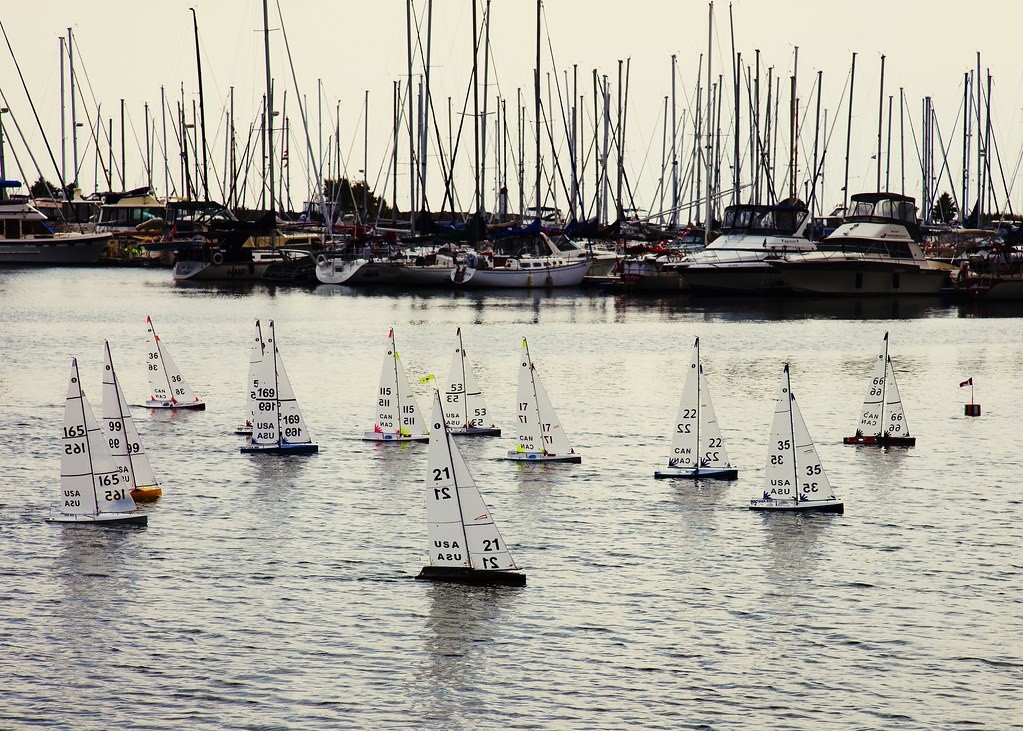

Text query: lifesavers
(212, 252), (223, 265)
(465, 253), (479, 268)
(316, 254), (326, 266)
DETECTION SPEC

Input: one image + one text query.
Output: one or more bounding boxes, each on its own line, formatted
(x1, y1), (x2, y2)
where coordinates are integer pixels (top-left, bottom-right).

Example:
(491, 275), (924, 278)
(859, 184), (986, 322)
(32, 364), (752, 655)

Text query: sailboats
(43, 356), (150, 529)
(240, 320), (319, 455)
(498, 334), (585, 468)
(131, 315), (207, 410)
(99, 337), (164, 502)
(841, 331), (916, 449)
(360, 325), (430, 443)
(435, 324), (503, 438)
(411, 374), (528, 588)
(0, 1), (1022, 301)
(234, 316), (266, 436)
(649, 335), (742, 481)
(748, 358), (846, 516)
